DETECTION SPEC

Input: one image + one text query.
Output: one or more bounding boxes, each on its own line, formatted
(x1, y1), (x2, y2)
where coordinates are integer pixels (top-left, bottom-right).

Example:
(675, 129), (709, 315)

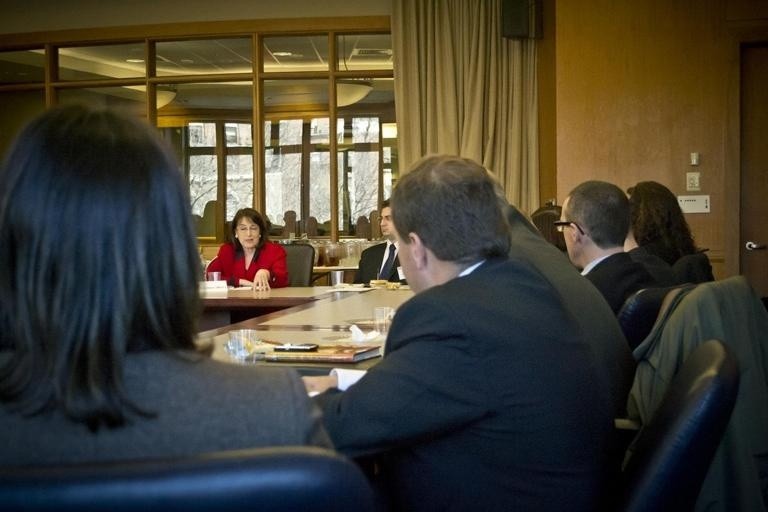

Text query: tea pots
(310, 238), (380, 267)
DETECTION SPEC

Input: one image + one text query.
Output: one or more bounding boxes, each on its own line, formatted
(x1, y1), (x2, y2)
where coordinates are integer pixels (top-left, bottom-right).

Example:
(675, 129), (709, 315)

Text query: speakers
(500, 0), (543, 39)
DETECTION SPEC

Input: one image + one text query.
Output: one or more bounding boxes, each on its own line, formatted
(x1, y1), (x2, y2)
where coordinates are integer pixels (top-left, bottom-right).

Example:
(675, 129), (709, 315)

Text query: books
(264, 345), (381, 364)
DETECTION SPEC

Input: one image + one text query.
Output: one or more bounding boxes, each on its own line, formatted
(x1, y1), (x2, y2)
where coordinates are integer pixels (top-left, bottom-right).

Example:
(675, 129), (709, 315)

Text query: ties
(379, 244), (396, 279)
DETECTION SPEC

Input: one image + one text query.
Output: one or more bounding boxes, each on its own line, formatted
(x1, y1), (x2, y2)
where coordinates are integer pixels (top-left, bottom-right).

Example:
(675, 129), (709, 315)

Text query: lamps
(155, 84), (178, 110)
(335, 80), (374, 108)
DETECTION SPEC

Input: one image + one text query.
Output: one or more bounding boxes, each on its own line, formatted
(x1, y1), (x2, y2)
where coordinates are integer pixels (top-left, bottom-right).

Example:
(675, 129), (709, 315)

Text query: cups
(331, 270), (344, 288)
(371, 308), (393, 336)
(207, 271), (221, 281)
(301, 232), (307, 240)
(227, 329), (258, 364)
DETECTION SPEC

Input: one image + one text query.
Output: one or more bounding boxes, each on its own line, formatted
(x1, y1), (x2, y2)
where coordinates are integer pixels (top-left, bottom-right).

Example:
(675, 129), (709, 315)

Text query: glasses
(554, 221), (585, 236)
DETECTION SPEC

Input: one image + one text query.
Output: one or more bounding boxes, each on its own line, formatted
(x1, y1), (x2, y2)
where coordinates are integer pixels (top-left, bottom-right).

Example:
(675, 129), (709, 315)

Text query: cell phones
(274, 344), (318, 352)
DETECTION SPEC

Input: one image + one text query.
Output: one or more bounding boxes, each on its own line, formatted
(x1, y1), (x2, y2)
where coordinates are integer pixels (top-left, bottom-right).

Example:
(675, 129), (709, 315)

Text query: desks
(313, 266), (358, 286)
(193, 284), (415, 370)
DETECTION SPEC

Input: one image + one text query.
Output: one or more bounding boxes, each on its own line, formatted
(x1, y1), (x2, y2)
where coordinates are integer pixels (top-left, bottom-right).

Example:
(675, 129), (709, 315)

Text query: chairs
(614, 275), (766, 512)
(0, 446), (378, 510)
(530, 206), (567, 251)
(279, 243), (315, 287)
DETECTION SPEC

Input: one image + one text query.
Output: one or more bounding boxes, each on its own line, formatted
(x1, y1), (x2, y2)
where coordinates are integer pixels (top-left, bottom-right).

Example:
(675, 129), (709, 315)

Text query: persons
(1, 102), (339, 478)
(554, 180), (658, 316)
(625, 180), (697, 282)
(207, 208), (289, 291)
(354, 198), (409, 285)
(486, 169), (638, 465)
(302, 153), (616, 511)
